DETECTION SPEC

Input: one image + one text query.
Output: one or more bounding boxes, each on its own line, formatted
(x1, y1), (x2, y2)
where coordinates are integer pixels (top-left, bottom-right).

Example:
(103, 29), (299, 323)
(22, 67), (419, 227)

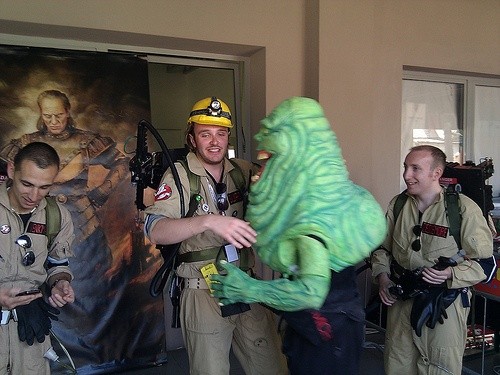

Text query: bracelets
(51, 279), (70, 290)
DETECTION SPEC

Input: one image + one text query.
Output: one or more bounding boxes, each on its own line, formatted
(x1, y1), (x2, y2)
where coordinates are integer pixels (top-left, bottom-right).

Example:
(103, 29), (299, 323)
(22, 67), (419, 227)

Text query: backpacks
(394, 160), (494, 250)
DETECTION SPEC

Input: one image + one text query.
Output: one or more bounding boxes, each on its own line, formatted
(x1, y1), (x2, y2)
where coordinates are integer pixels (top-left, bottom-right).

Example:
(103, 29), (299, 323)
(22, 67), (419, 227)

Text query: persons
(0, 142), (75, 375)
(208, 96), (388, 375)
(370, 145), (496, 375)
(143, 99), (289, 375)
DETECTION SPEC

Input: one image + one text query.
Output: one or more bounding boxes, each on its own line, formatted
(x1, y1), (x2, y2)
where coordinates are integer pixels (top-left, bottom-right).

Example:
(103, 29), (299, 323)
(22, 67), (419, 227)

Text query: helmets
(187, 97), (232, 128)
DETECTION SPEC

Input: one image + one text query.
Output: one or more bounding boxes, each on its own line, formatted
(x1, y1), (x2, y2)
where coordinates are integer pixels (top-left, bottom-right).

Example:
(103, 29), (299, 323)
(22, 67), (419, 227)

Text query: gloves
(410, 288), (460, 335)
(16, 288), (60, 345)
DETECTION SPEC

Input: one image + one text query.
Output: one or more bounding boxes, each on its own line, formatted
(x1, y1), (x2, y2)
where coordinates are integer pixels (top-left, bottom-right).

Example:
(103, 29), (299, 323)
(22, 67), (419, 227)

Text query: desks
(462, 276), (500, 375)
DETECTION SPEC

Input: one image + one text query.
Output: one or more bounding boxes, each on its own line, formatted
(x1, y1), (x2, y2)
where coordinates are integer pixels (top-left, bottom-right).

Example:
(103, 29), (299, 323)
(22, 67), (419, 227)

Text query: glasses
(216, 181), (229, 212)
(412, 224), (422, 252)
(15, 235), (35, 267)
